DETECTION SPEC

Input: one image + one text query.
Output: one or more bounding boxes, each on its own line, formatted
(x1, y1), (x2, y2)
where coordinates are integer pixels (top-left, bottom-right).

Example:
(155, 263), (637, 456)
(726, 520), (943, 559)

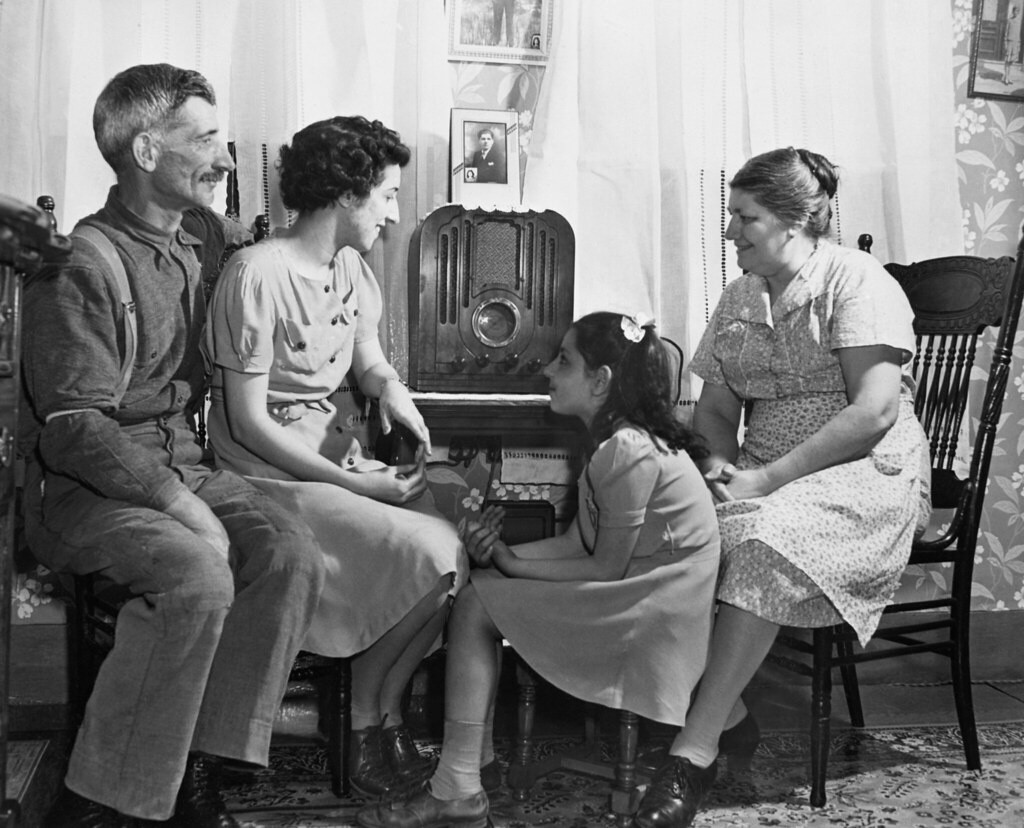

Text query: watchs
(380, 378), (408, 393)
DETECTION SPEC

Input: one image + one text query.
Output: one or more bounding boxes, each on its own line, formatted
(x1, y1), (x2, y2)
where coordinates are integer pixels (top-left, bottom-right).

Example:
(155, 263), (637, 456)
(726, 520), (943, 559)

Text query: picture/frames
(451, 108), (521, 206)
(966, 0), (1024, 103)
(444, 0), (557, 65)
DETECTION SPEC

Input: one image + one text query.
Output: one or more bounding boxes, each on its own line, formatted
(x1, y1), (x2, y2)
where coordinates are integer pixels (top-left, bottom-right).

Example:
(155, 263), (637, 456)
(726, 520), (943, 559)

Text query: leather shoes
(634, 749), (718, 828)
(39, 784), (133, 828)
(349, 713), (398, 801)
(634, 708), (761, 774)
(419, 754), (506, 795)
(383, 722), (425, 783)
(175, 754), (242, 828)
(354, 778), (490, 828)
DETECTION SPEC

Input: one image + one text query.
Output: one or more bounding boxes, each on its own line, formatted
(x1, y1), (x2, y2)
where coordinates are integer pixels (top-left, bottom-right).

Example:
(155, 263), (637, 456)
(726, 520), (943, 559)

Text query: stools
(505, 655), (676, 828)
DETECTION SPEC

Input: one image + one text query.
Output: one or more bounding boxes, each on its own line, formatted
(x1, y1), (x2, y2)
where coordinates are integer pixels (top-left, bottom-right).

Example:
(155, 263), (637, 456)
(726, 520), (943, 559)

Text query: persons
(356, 312), (720, 828)
(633, 146), (933, 828)
(490, 0), (515, 47)
(467, 129), (507, 184)
(467, 170), (475, 182)
(18, 63), (324, 828)
(1003, 6), (1021, 85)
(197, 114), (470, 799)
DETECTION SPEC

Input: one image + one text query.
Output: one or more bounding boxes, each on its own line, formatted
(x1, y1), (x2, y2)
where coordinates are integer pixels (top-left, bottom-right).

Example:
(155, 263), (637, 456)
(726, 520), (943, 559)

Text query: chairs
(770, 227), (1024, 808)
(61, 570), (355, 828)
